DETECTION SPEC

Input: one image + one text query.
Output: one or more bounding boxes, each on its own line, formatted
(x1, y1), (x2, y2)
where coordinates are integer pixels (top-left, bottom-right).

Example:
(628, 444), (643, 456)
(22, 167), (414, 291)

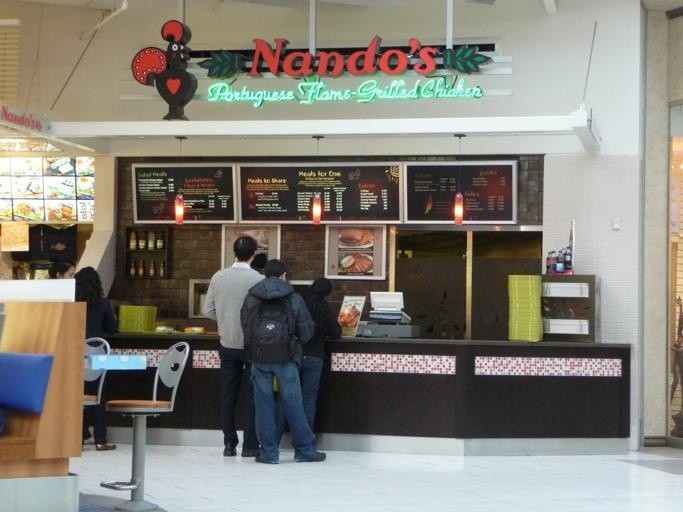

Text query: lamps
(1, 140), (29, 252)
(312, 135), (325, 226)
(453, 134), (466, 226)
(174, 136), (187, 225)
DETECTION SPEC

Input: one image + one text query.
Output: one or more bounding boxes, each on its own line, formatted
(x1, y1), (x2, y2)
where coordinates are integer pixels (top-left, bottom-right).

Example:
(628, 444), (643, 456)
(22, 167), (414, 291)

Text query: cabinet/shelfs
(123, 226), (170, 280)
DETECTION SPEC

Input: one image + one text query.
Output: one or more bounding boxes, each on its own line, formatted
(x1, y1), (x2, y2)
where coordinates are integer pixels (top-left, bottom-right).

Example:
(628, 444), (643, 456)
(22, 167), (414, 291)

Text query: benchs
(0, 301), (88, 512)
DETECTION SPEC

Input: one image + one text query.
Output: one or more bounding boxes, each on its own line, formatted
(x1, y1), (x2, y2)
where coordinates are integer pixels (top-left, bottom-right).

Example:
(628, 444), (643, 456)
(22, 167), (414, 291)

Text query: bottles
(147, 260), (155, 277)
(129, 259), (136, 277)
(183, 327), (205, 335)
(128, 230), (137, 250)
(147, 230), (154, 250)
(138, 231), (146, 249)
(158, 261), (165, 277)
(545, 246), (571, 274)
(156, 232), (164, 249)
(137, 259), (144, 276)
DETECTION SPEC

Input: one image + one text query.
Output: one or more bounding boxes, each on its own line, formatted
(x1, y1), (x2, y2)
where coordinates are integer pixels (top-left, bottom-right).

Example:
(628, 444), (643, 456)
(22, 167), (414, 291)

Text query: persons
(44, 254), (75, 278)
(241, 259), (327, 464)
(202, 236), (265, 458)
(73, 265), (116, 450)
(272, 278), (342, 451)
(249, 252), (267, 275)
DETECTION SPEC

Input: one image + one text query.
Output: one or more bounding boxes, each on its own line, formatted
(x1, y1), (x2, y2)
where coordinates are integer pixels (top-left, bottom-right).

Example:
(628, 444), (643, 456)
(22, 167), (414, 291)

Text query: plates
(338, 254), (374, 273)
(117, 305), (157, 332)
(506, 274), (543, 343)
(338, 234), (373, 249)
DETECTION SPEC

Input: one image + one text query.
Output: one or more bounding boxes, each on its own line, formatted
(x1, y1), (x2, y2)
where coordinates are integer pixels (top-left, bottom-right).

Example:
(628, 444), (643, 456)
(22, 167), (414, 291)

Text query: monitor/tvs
(370, 292), (404, 312)
(288, 280), (315, 293)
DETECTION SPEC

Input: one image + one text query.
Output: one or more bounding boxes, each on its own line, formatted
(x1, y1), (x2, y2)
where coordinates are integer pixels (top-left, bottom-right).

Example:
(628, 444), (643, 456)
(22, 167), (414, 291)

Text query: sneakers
(294, 452), (326, 462)
(96, 444), (116, 450)
(242, 448), (261, 457)
(255, 458), (280, 463)
(224, 442), (235, 455)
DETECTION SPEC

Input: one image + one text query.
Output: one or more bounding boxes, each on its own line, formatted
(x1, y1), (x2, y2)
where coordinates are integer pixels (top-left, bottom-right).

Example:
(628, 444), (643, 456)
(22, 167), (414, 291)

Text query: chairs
(100, 341), (190, 511)
(83, 337), (111, 406)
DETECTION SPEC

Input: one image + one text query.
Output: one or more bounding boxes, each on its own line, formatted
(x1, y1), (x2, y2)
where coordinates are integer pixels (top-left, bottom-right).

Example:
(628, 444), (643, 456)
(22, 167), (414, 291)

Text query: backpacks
(244, 298), (296, 363)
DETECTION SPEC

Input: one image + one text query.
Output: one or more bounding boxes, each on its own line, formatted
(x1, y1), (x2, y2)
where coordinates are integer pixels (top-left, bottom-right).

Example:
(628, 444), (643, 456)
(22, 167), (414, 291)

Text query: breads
(62, 205), (72, 218)
(339, 229), (372, 246)
(348, 259), (374, 272)
(19, 204), (30, 212)
(338, 306), (359, 328)
